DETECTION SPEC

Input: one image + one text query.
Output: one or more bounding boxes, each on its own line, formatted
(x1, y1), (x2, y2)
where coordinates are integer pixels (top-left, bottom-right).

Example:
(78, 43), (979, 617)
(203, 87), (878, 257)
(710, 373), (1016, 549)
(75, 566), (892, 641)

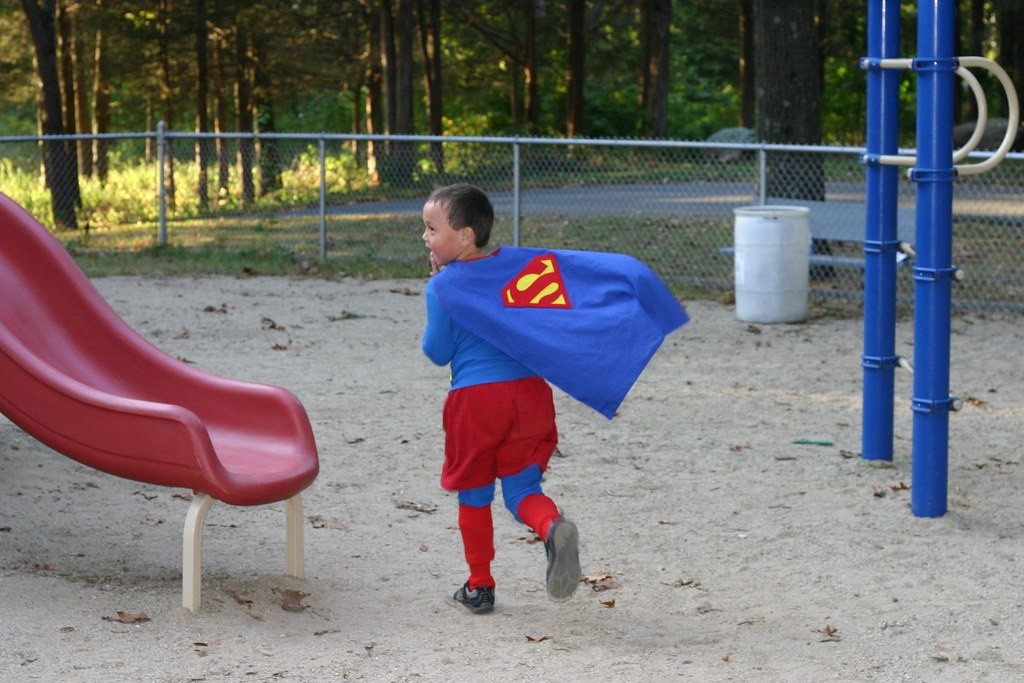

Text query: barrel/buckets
(731, 205), (811, 324)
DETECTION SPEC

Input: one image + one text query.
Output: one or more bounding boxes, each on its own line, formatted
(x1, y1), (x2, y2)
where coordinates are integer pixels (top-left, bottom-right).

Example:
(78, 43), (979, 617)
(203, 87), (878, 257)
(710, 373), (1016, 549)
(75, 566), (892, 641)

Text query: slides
(0, 191), (321, 507)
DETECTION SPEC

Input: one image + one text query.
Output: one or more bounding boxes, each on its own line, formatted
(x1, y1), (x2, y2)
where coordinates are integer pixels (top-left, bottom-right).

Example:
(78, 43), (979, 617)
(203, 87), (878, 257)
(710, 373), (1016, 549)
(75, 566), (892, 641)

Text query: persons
(422, 183), (691, 615)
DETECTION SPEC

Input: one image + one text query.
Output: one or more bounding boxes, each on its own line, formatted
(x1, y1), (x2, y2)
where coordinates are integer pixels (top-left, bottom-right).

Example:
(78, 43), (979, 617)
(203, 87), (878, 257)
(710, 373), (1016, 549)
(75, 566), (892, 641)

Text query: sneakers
(452, 579), (495, 614)
(544, 515), (580, 603)
